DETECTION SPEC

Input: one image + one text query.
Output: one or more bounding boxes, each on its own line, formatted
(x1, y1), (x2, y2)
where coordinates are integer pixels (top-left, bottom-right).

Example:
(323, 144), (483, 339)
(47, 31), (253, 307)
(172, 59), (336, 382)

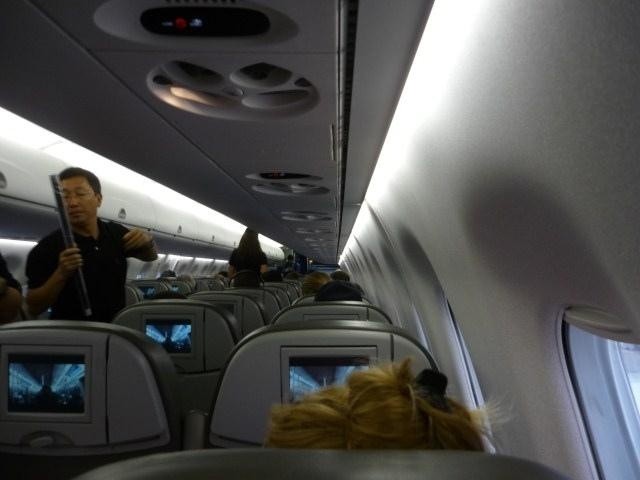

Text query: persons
(266, 355), (501, 454)
(280, 253), (302, 280)
(228, 226), (268, 287)
(317, 280), (364, 303)
(287, 272), (300, 280)
(23, 167), (159, 326)
(262, 269), (284, 282)
(330, 269), (351, 282)
(302, 271), (331, 296)
(0, 171), (24, 333)
